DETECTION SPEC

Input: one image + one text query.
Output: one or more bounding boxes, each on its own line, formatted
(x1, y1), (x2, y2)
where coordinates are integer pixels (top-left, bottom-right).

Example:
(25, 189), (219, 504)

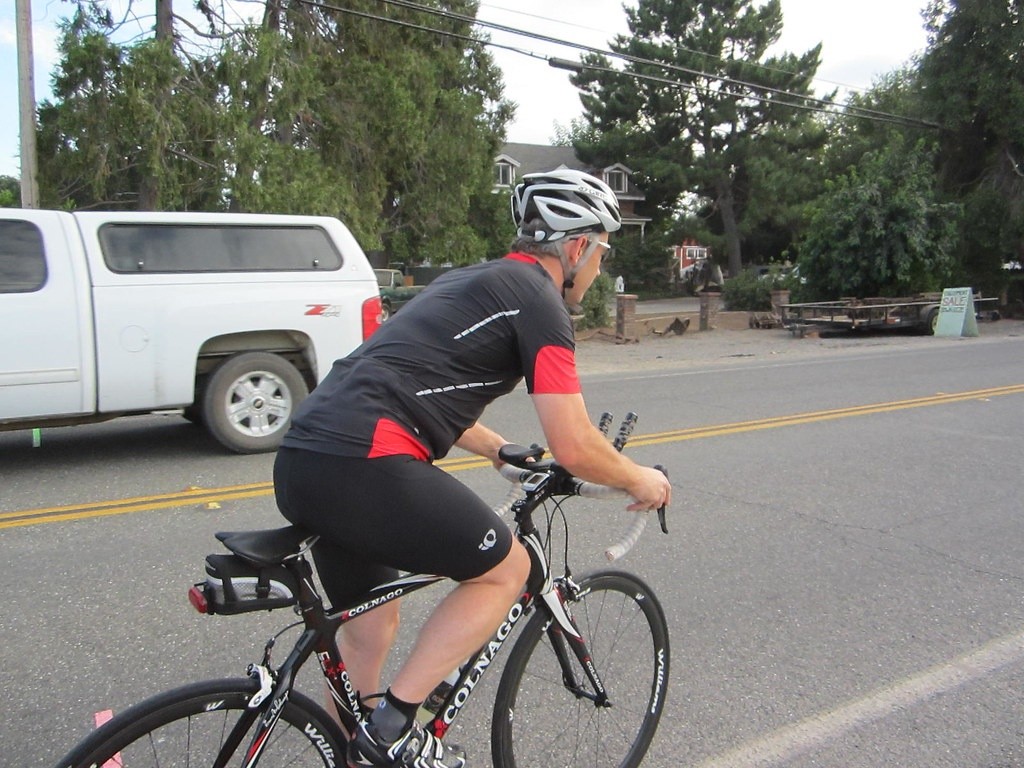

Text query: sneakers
(347, 716), (466, 768)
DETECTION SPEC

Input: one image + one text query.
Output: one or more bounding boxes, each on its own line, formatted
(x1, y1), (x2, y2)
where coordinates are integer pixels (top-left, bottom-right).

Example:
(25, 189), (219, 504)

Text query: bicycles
(53, 410), (670, 768)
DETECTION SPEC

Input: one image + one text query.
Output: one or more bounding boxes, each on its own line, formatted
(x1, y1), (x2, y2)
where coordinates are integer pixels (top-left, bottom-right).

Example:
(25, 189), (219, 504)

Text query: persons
(271, 166), (673, 768)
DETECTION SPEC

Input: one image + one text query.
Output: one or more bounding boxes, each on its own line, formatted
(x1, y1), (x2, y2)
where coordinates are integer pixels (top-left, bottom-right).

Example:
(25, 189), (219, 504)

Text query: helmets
(508, 167), (623, 240)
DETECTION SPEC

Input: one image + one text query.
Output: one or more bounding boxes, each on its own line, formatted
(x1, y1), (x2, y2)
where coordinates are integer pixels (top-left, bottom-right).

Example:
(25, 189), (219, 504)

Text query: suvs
(0, 209), (389, 452)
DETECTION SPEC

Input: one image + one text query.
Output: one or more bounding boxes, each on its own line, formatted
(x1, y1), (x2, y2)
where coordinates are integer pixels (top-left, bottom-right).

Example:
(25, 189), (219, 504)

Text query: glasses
(581, 233), (612, 264)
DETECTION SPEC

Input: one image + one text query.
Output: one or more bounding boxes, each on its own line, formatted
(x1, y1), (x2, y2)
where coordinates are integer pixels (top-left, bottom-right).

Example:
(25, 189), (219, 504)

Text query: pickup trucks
(373, 269), (428, 323)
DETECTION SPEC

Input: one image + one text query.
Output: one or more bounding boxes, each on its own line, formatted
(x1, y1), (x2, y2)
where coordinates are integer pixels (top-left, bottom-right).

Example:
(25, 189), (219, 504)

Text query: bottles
(412, 659), (465, 728)
